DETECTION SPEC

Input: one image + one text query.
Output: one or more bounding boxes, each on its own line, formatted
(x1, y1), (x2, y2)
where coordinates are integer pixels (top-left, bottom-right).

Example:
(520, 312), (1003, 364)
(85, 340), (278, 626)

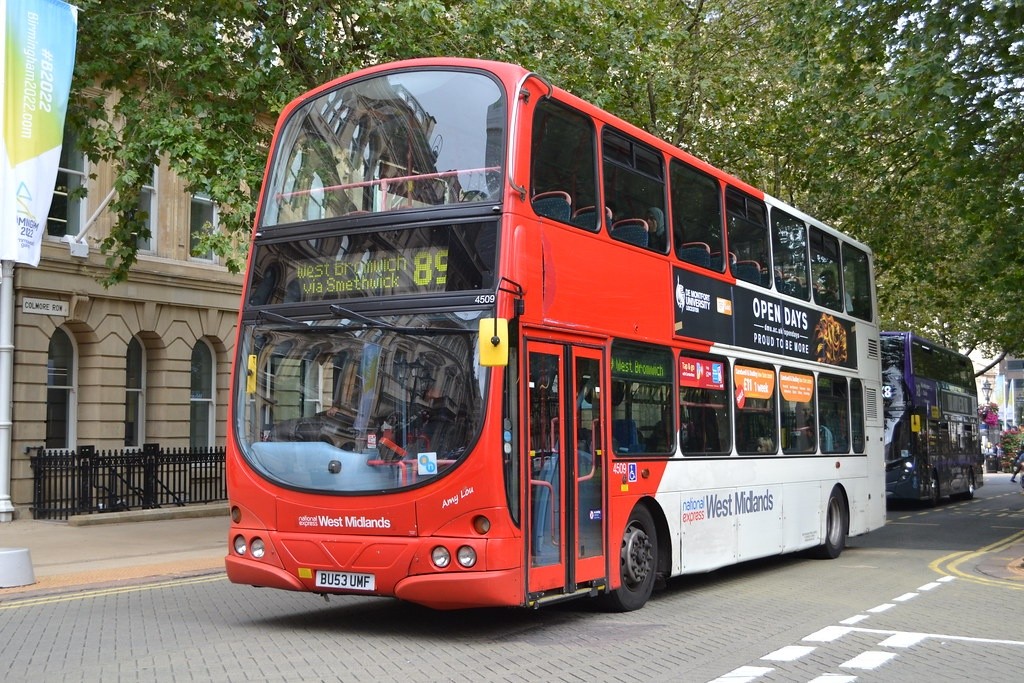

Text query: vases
(1004, 466), (1010, 473)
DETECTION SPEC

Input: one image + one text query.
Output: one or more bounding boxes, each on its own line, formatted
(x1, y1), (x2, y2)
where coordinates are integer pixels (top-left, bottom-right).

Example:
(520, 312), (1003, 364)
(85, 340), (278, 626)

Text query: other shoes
(1010, 478), (1017, 483)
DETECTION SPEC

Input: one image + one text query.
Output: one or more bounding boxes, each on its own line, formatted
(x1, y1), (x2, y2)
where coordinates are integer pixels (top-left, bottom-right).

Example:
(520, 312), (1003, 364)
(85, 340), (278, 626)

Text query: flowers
(998, 428), (1021, 467)
(978, 403), (999, 427)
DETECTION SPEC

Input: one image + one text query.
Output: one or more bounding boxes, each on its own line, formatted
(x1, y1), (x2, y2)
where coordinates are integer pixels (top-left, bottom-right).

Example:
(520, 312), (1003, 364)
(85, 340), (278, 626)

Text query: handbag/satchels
(1013, 456), (1019, 466)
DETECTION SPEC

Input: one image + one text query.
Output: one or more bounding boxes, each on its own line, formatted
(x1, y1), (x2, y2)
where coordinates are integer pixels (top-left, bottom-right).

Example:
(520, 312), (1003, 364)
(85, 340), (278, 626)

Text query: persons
(327, 393), (378, 426)
(981, 442), (1024, 483)
(646, 206), (667, 250)
(656, 390), (822, 455)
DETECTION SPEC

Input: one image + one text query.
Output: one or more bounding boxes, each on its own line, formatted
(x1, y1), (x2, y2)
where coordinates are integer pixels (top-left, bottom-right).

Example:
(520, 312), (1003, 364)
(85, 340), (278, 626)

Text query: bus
(880, 329), (987, 508)
(223, 56), (888, 615)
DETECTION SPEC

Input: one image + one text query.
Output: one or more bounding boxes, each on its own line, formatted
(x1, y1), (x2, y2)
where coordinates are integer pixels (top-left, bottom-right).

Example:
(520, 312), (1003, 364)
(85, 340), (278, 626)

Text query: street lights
(982, 379), (994, 407)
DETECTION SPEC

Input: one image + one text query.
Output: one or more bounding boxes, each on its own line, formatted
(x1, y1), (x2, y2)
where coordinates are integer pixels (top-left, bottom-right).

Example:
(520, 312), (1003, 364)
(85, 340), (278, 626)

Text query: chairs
(531, 191), (838, 311)
(820, 425), (833, 452)
(611, 419), (639, 454)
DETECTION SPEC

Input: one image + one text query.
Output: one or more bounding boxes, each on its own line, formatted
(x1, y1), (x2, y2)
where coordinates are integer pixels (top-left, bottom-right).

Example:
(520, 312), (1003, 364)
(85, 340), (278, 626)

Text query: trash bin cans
(986, 454), (997, 473)
